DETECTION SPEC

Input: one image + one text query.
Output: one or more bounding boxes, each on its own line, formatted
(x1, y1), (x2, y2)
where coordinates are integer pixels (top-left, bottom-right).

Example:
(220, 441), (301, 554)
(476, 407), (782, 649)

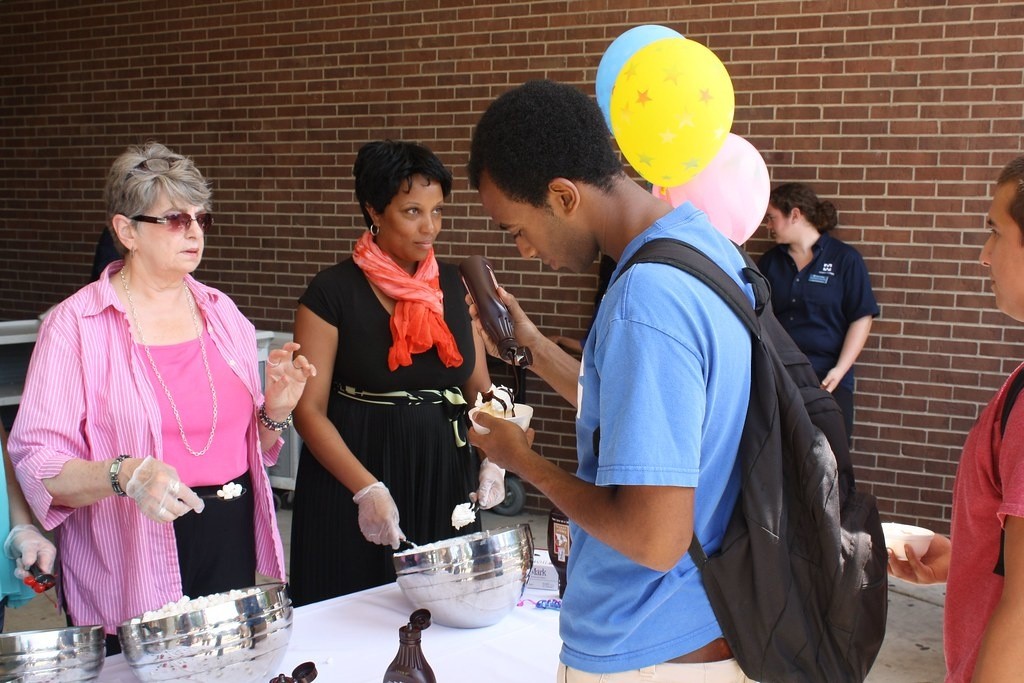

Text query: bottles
(383, 609), (436, 683)
(269, 660), (317, 683)
(547, 507), (572, 599)
(458, 255), (533, 370)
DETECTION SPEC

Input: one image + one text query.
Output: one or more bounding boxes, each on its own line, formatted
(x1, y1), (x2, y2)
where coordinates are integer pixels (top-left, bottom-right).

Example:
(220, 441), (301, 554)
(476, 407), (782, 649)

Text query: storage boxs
(529, 549), (560, 590)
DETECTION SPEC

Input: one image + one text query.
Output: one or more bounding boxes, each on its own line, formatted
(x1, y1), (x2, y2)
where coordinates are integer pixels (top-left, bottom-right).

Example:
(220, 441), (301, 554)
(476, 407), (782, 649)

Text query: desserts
(474, 383), (515, 420)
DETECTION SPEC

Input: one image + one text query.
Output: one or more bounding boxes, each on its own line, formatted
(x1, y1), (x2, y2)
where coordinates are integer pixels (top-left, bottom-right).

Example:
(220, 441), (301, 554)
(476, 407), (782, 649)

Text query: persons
(883, 157), (1024, 683)
(761, 183), (876, 445)
(0, 141), (318, 658)
(287, 137), (506, 610)
(470, 79), (764, 683)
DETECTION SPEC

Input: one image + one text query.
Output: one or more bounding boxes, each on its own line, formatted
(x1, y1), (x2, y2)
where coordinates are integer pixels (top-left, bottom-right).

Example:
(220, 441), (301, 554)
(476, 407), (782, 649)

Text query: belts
(663, 638), (734, 663)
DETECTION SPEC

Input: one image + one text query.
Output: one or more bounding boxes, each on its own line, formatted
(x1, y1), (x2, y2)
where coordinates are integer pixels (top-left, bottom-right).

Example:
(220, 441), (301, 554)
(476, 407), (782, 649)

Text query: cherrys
(24, 574), (56, 593)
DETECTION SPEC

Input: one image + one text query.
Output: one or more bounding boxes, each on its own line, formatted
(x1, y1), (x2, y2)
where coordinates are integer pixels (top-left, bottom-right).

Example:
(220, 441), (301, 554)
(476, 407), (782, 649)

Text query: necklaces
(120, 265), (217, 455)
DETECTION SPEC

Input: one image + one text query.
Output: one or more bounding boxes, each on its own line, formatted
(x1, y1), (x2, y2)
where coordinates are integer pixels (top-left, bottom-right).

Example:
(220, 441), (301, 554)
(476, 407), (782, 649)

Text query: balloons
(593, 24), (775, 246)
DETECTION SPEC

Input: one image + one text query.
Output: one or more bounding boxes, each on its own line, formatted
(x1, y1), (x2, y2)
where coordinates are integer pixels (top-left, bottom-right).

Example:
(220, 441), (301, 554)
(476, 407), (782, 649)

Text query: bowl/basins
(468, 403), (533, 436)
(116, 582), (294, 683)
(0, 626), (107, 683)
(393, 523), (534, 629)
(881, 522), (935, 562)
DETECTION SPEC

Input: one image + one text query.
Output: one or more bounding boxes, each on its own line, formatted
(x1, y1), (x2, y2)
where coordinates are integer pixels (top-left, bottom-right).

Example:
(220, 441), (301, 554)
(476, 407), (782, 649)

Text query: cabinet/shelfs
(0, 319), (302, 510)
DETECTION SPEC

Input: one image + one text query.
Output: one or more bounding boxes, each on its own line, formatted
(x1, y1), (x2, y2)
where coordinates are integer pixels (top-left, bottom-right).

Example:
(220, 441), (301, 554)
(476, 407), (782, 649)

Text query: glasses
(131, 213), (214, 234)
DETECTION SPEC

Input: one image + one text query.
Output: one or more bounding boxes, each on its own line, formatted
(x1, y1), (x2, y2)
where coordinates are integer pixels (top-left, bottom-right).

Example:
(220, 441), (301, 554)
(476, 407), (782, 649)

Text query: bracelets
(258, 402), (292, 431)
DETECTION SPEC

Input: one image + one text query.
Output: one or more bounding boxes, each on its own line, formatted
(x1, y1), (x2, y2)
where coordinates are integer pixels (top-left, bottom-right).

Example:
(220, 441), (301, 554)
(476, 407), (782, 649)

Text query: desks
(90, 551), (564, 683)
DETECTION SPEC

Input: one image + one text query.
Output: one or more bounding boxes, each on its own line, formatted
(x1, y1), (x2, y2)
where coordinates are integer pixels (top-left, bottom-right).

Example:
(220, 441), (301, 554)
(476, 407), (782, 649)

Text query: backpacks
(592, 237), (888, 683)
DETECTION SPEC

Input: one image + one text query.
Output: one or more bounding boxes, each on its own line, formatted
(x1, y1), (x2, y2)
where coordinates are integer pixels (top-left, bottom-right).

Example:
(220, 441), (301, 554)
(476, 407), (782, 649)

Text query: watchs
(109, 454), (132, 496)
